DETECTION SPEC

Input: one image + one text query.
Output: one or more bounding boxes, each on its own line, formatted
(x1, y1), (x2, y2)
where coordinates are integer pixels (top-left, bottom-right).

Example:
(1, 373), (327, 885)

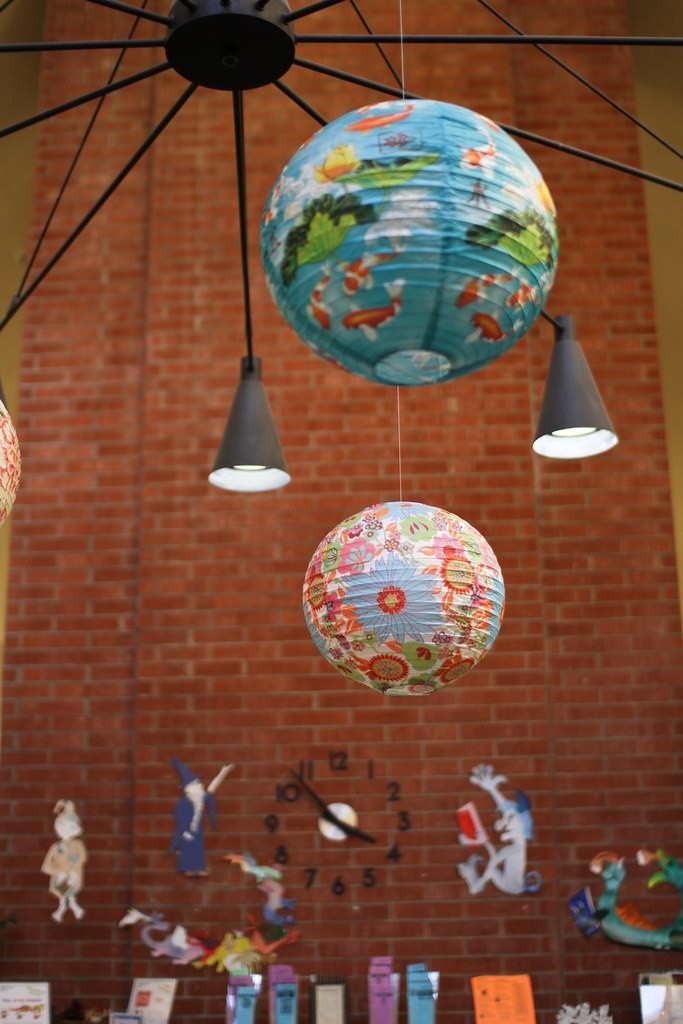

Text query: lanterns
(303, 502), (506, 698)
(260, 98), (560, 392)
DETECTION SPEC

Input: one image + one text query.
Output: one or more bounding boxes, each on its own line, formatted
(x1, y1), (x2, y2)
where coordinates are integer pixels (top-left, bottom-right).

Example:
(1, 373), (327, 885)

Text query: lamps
(1, 0), (683, 497)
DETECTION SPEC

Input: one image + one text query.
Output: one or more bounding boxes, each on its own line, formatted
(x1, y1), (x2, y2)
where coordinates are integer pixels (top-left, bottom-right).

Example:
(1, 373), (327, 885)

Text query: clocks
(261, 748), (410, 898)
